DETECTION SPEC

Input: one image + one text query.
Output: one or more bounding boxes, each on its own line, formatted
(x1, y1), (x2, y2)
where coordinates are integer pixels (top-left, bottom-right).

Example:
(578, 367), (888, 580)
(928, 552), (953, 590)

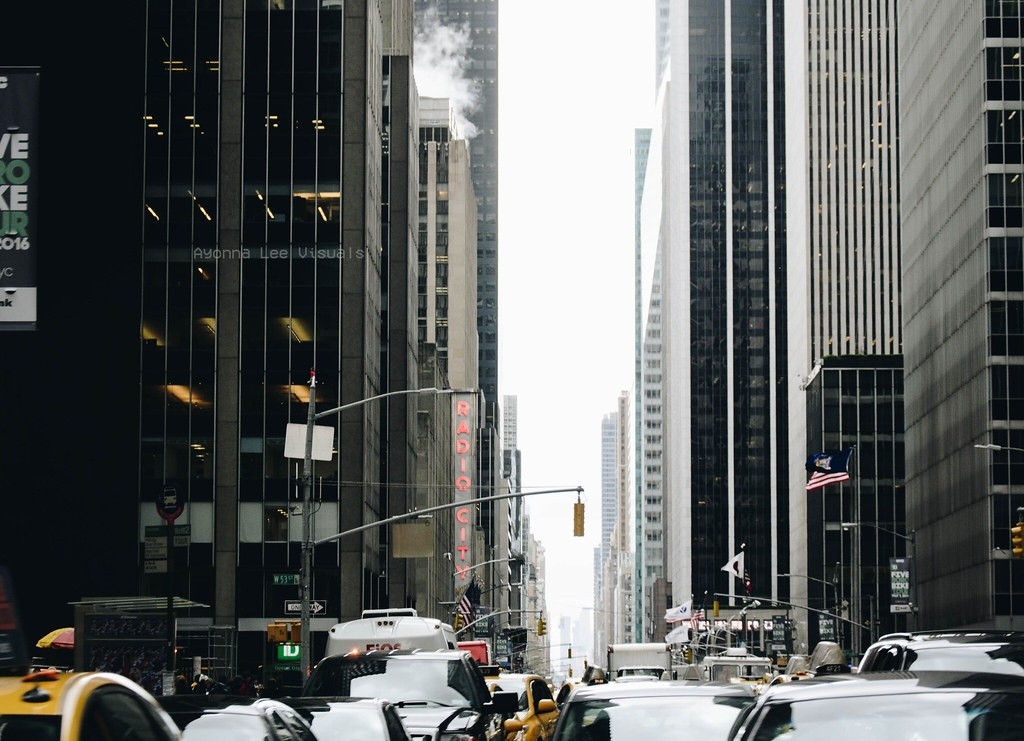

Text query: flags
(665, 625), (690, 644)
(690, 596), (705, 629)
(457, 580), (476, 633)
(804, 447), (853, 473)
(720, 551), (744, 579)
(664, 600), (692, 623)
(805, 470), (849, 491)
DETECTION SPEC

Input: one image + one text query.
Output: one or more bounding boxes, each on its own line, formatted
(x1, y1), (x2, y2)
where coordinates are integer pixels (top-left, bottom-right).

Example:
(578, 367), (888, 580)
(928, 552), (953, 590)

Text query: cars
(727, 674), (1024, 741)
(557, 666), (617, 725)
(158, 695), (319, 740)
(273, 694), (412, 741)
(0, 671), (184, 741)
(552, 682), (755, 741)
(479, 664), (560, 740)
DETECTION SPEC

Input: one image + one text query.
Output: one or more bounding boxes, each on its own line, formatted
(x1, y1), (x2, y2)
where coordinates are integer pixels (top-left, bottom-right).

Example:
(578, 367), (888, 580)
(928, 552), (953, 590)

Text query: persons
(189, 674), (231, 695)
(239, 676), (279, 699)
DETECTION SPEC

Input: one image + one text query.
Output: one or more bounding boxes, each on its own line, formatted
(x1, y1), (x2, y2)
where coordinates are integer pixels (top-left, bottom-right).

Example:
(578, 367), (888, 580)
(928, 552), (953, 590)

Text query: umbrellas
(36, 627), (74, 648)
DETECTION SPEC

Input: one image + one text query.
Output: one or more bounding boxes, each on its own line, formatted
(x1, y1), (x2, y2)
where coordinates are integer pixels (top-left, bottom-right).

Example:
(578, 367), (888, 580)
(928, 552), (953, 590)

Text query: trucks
(608, 643), (678, 681)
(325, 606), (459, 657)
(705, 647), (771, 682)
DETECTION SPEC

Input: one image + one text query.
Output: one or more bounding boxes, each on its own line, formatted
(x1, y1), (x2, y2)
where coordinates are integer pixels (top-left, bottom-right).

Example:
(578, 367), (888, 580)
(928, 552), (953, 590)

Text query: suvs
(306, 650), (520, 740)
(857, 631), (1024, 680)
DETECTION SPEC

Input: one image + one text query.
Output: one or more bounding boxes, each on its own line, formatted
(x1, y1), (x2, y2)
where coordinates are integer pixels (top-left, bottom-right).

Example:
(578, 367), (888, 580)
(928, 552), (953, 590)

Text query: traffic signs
(284, 600), (325, 616)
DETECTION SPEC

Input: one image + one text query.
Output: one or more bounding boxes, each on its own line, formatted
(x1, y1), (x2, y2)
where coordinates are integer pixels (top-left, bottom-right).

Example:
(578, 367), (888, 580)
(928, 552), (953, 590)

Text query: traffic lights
(685, 650), (692, 662)
(1009, 524), (1023, 556)
(568, 668), (572, 677)
(538, 619), (548, 636)
(454, 613), (466, 630)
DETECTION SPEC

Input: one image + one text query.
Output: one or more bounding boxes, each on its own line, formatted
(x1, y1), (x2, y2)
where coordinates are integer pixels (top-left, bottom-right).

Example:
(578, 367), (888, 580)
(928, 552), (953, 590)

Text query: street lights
(695, 623), (740, 650)
(297, 386), (439, 682)
(494, 616), (557, 682)
(777, 574), (842, 649)
(448, 555), (526, 626)
(472, 581), (523, 642)
(841, 522), (919, 631)
